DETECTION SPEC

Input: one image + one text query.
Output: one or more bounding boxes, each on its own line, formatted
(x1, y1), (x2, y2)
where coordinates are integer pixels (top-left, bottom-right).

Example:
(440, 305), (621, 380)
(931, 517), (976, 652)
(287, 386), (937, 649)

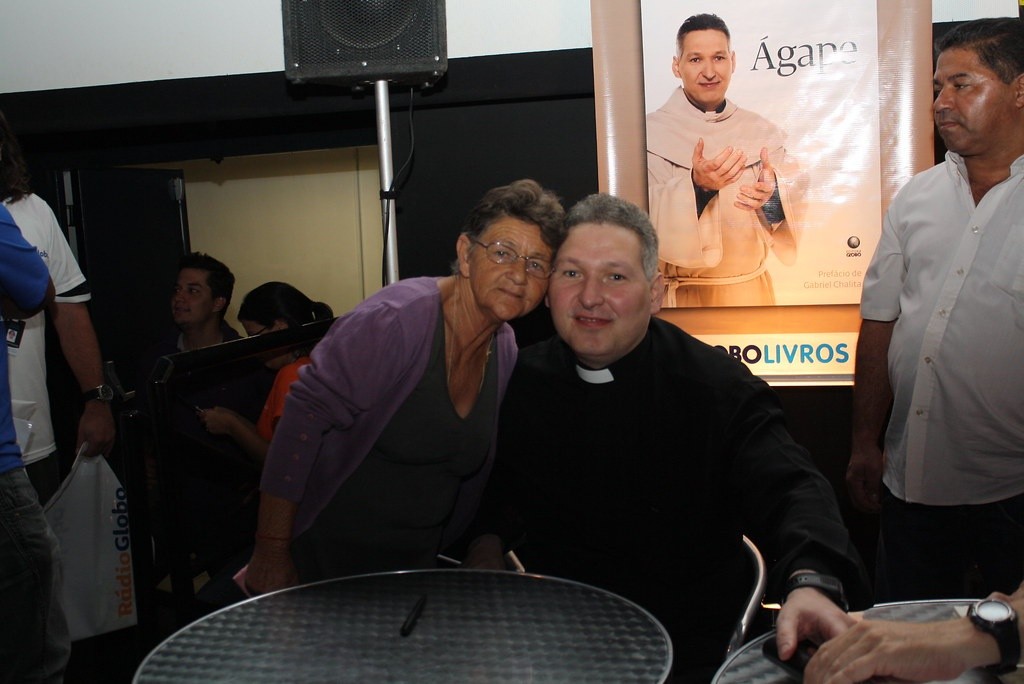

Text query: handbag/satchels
(42, 443), (138, 643)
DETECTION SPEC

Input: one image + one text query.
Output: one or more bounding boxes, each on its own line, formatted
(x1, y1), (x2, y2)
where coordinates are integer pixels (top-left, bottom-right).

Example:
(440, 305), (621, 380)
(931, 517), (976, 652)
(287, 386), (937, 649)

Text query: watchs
(967, 599), (1021, 675)
(84, 384), (113, 402)
(783, 573), (849, 614)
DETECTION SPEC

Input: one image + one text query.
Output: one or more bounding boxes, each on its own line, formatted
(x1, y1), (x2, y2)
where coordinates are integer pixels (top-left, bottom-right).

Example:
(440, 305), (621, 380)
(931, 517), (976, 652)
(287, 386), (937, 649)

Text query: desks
(132, 569), (674, 684)
(712, 598), (1004, 684)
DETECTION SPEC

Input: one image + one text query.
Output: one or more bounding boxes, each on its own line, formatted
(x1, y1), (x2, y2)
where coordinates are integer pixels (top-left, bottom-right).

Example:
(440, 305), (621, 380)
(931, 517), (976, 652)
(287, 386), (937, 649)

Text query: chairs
(501, 534), (766, 658)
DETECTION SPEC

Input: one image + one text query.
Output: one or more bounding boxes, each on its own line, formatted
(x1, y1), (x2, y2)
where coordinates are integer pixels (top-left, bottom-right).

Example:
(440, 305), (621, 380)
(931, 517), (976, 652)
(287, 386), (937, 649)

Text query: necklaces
(447, 279), (495, 395)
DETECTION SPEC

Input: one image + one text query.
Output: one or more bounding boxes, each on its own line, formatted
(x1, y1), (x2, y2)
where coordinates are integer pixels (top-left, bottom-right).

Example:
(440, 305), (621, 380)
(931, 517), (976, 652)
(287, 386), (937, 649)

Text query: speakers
(282, 0), (448, 93)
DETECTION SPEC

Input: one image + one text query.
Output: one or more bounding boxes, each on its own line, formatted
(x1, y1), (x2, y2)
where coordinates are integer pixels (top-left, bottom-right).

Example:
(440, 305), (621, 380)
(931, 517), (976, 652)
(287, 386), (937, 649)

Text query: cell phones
(194, 405), (206, 413)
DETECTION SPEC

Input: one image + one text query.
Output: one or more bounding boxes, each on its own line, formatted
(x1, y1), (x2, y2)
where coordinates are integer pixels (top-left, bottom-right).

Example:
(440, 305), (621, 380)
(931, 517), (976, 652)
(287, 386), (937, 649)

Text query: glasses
(472, 238), (556, 279)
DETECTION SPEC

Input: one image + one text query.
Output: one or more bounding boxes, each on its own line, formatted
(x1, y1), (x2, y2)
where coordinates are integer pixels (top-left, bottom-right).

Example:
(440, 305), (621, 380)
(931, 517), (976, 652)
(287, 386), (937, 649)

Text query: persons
(846, 17), (1024, 603)
(0, 112), (116, 505)
(142, 252), (275, 620)
(194, 282), (335, 465)
(246, 178), (568, 596)
(803, 598), (1024, 684)
(465, 193), (857, 661)
(0, 204), (72, 684)
(646, 14), (810, 309)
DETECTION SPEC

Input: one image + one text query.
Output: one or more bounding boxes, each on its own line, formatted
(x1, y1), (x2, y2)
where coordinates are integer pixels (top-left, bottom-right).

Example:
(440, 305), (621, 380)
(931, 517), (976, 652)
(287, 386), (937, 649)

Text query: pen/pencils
(399, 593), (429, 637)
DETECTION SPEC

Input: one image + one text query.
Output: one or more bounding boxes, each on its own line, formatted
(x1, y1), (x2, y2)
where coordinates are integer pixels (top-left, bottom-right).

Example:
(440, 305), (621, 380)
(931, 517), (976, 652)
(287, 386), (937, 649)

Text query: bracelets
(255, 533), (289, 542)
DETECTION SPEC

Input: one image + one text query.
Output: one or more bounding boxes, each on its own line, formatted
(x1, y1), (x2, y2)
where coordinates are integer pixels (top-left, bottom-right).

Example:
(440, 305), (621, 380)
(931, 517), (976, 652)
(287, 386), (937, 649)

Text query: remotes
(762, 635), (887, 684)
